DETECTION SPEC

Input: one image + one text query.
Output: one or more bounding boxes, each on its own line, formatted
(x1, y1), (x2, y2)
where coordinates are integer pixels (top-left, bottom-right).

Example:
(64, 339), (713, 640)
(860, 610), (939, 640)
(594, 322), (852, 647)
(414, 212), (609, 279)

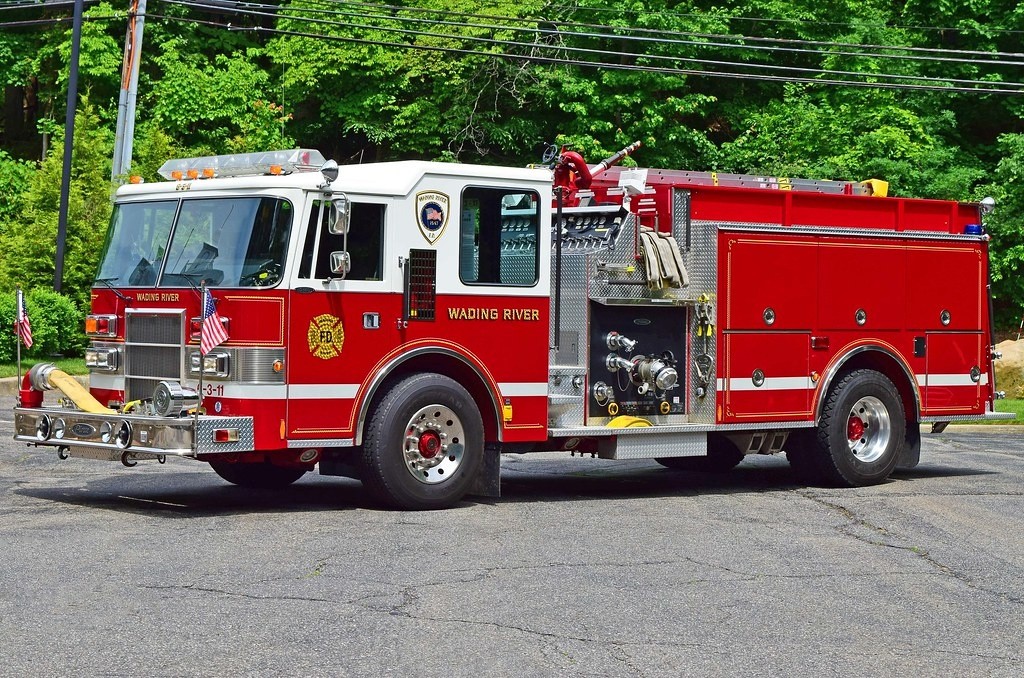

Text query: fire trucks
(12, 141), (1017, 513)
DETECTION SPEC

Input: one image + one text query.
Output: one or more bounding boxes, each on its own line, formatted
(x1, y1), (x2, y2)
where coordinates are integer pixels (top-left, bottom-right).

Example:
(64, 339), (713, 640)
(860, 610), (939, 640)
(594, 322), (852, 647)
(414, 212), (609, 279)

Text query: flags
(201, 288), (230, 358)
(15, 290), (34, 349)
